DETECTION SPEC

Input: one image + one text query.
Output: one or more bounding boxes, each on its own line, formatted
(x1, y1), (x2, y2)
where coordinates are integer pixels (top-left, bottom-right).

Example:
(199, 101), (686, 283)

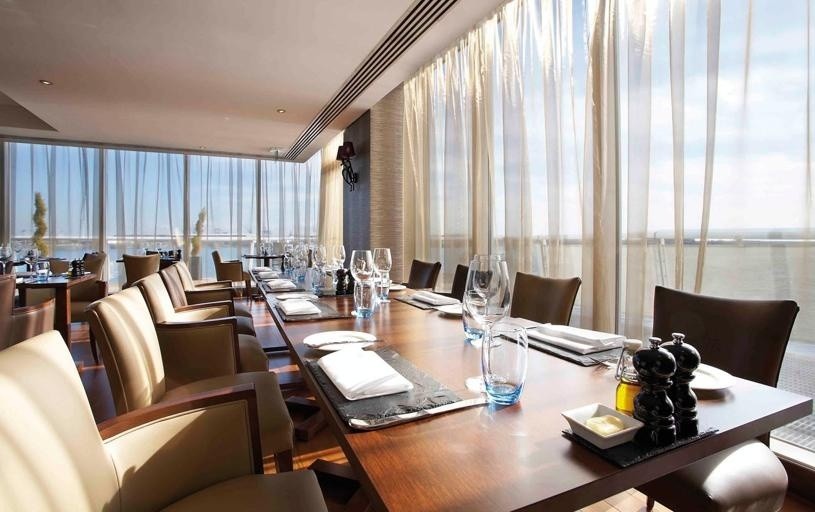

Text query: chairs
(70, 251), (110, 365)
(436, 263), (492, 305)
(0, 329), (331, 512)
(83, 286), (296, 472)
(133, 273), (269, 372)
(510, 271), (583, 328)
(175, 260), (252, 316)
(629, 284), (799, 512)
(158, 264), (257, 337)
(0, 274), (54, 349)
(120, 254), (161, 291)
(391, 258), (441, 292)
(211, 250), (253, 300)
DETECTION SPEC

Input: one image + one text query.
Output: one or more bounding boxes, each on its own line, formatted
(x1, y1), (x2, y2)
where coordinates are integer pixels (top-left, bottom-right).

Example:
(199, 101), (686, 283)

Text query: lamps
(336, 141), (360, 191)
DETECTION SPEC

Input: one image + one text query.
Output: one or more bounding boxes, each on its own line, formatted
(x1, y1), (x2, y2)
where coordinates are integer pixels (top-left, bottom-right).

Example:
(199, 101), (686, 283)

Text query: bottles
(616, 339), (642, 419)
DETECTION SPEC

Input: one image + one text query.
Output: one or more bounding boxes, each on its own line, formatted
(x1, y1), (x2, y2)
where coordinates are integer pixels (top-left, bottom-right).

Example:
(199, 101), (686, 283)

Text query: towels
(317, 346), (415, 396)
(257, 271), (278, 278)
(414, 289), (460, 309)
(275, 301), (322, 317)
(267, 279), (297, 290)
(252, 266), (274, 274)
(525, 322), (626, 354)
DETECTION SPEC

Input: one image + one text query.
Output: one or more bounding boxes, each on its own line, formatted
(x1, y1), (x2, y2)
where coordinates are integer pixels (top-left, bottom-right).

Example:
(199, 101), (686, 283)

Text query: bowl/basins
(561, 402), (644, 451)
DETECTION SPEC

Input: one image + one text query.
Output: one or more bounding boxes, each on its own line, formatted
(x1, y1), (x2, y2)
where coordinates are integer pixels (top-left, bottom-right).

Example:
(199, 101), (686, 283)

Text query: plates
(277, 293), (318, 300)
(264, 279), (293, 283)
(688, 363), (737, 391)
(303, 330), (378, 352)
(61, 272), (91, 278)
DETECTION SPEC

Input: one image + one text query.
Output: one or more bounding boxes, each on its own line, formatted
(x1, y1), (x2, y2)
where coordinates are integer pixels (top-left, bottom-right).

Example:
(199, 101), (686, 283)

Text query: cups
(36, 262), (51, 281)
(462, 289), (509, 347)
(480, 322), (528, 407)
(374, 272), (390, 300)
(354, 278), (375, 316)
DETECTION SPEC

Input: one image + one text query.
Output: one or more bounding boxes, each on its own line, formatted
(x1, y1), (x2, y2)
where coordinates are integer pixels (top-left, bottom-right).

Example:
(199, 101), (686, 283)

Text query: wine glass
(283, 244), (348, 298)
(373, 248), (392, 303)
(157, 242), (162, 258)
(464, 260), (514, 349)
(473, 254), (500, 337)
(143, 242), (149, 255)
(24, 248), (39, 283)
(350, 250), (374, 318)
(0, 246), (14, 276)
